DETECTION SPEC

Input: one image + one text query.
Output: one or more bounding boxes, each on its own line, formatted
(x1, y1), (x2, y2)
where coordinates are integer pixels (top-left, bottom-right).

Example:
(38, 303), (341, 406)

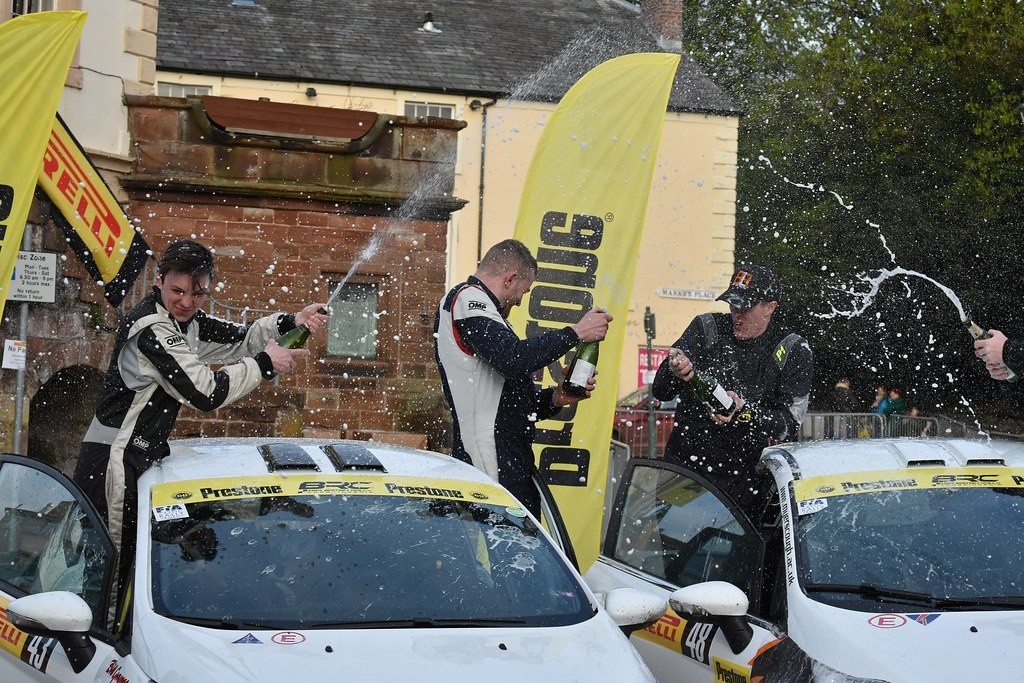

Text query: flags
(510, 51), (683, 574)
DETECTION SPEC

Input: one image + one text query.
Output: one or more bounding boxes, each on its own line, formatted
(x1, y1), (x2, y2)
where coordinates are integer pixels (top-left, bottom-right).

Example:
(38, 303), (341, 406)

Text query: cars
(0, 436), (668, 683)
(612, 384), (679, 459)
(584, 438), (1024, 683)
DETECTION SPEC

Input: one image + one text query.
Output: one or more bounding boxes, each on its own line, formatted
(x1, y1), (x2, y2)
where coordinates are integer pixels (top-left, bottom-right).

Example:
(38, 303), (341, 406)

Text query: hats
(716, 263), (785, 312)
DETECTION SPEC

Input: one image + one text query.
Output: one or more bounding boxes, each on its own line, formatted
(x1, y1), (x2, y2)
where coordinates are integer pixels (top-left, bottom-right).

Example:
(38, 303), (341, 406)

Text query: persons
(651, 265), (815, 595)
(71, 239), (337, 637)
(428, 238), (615, 586)
(832, 374), (936, 440)
(973, 323), (1024, 407)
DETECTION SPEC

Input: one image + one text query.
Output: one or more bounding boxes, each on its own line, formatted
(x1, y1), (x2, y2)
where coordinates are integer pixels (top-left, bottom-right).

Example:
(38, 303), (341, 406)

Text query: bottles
(961, 317), (1019, 383)
(673, 352), (737, 417)
(262, 307), (328, 382)
(562, 312), (607, 397)
